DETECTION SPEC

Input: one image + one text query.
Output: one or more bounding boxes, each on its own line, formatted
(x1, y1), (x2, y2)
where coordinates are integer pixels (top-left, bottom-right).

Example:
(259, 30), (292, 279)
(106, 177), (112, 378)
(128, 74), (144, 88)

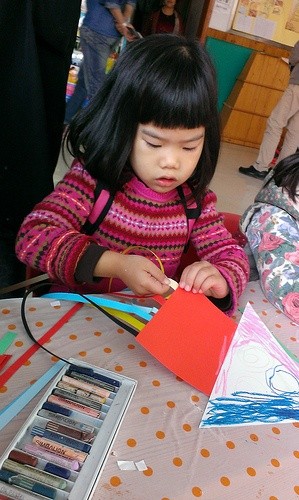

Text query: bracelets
(120, 22), (128, 27)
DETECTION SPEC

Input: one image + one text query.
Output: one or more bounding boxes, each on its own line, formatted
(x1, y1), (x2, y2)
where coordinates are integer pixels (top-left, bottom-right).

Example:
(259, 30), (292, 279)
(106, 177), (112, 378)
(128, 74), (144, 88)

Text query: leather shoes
(239, 165), (272, 181)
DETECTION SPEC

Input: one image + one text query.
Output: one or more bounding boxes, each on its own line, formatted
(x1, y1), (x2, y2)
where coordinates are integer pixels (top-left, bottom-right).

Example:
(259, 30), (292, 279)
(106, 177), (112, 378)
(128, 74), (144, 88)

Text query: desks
(0, 280), (299, 500)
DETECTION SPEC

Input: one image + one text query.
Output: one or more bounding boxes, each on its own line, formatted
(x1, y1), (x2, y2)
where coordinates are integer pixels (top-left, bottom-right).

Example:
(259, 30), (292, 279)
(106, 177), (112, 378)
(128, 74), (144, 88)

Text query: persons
(16, 34), (250, 318)
(239, 40), (299, 179)
(234, 152), (299, 325)
(142, 0), (182, 35)
(63, 0), (141, 126)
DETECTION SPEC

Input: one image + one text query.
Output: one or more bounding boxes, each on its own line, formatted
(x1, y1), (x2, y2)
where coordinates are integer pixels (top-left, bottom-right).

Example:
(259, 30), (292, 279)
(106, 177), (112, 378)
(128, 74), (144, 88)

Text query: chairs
(25, 211), (243, 288)
